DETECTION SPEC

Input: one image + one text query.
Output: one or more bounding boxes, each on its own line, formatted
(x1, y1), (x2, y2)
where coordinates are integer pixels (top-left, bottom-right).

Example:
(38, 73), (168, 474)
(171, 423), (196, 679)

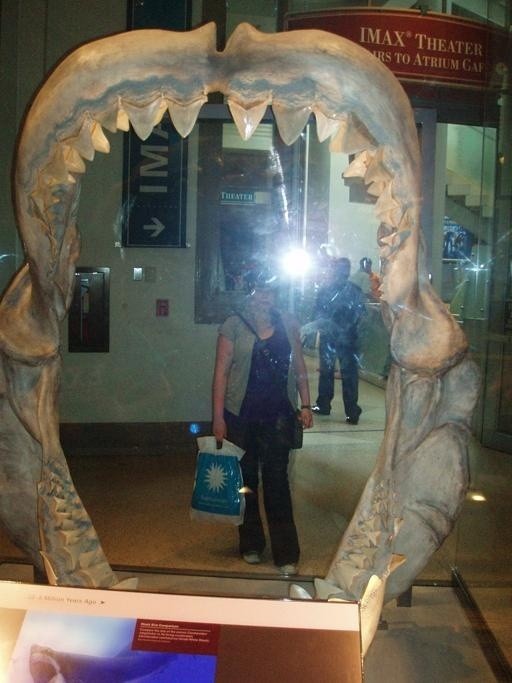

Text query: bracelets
(302, 405), (311, 409)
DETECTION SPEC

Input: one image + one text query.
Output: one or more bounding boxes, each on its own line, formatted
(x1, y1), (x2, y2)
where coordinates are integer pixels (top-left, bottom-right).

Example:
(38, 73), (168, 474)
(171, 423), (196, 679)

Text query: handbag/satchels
(191, 437), (249, 526)
(292, 413), (303, 450)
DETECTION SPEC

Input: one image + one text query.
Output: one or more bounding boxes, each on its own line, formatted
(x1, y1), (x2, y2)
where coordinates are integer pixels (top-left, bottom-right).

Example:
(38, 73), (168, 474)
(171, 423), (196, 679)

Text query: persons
(315, 258), (363, 424)
(211, 270), (314, 575)
(368, 271), (381, 303)
(352, 257), (371, 295)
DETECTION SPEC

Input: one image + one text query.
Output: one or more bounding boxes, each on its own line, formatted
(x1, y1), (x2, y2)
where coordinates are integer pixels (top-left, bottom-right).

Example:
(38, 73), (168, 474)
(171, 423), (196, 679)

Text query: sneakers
(244, 551), (260, 564)
(346, 416), (357, 425)
(311, 405), (330, 414)
(280, 563), (299, 574)
